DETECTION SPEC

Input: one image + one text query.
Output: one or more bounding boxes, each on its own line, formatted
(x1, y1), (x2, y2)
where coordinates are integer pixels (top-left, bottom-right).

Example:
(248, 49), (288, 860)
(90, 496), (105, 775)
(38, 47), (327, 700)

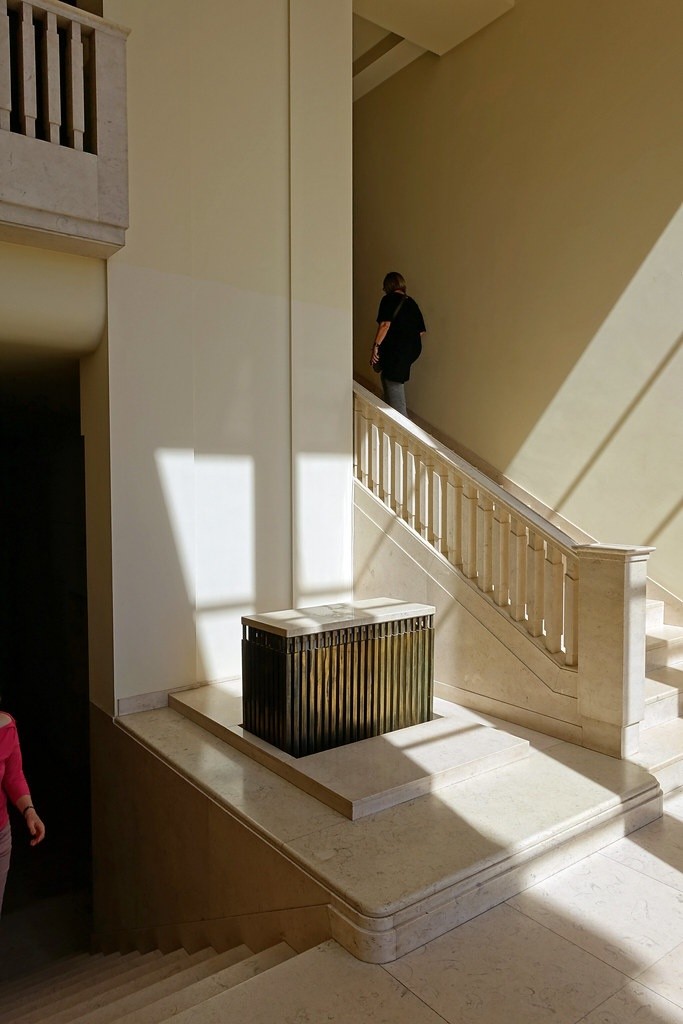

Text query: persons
(0, 710), (45, 910)
(370, 272), (426, 417)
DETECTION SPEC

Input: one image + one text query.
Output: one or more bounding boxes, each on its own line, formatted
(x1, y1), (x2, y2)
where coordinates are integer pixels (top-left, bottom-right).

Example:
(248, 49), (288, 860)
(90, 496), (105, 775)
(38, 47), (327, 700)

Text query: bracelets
(374, 342), (380, 347)
(23, 806), (34, 817)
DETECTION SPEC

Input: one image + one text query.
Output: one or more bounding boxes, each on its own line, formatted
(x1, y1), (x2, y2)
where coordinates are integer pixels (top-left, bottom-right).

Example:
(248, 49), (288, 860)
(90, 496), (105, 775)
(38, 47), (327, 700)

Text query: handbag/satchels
(373, 348), (382, 373)
